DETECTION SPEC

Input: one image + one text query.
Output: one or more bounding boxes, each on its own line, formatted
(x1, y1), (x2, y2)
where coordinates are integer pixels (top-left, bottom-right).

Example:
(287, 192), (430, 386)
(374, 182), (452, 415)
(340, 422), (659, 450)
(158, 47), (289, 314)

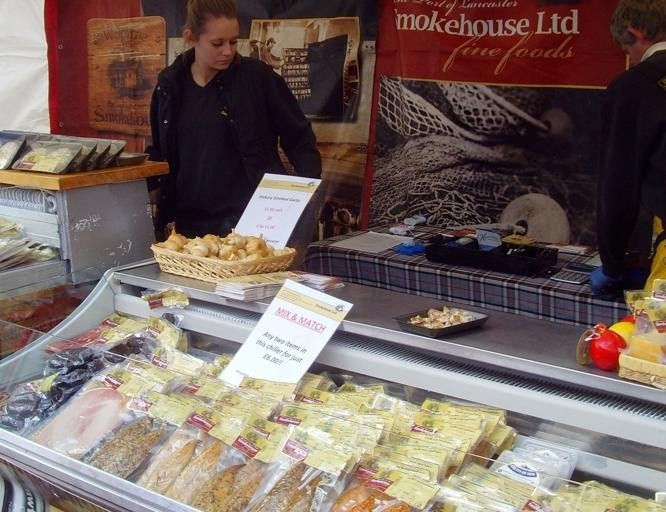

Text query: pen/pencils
(407, 229), (425, 238)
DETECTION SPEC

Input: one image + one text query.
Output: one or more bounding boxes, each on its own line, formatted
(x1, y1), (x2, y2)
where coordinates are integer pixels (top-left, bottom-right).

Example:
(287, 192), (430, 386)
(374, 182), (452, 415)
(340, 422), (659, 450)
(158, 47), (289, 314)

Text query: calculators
(550, 262), (598, 285)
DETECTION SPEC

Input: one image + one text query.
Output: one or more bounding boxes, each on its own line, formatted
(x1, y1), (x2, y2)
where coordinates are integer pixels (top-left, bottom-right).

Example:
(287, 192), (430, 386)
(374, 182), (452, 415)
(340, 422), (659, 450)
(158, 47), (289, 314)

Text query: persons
(586, 0), (665, 301)
(256, 36), (289, 71)
(140, 0), (323, 245)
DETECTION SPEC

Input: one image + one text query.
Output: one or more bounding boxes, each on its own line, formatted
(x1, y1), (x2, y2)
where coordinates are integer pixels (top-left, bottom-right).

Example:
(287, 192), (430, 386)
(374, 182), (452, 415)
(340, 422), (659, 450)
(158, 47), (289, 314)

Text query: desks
(306, 221), (632, 330)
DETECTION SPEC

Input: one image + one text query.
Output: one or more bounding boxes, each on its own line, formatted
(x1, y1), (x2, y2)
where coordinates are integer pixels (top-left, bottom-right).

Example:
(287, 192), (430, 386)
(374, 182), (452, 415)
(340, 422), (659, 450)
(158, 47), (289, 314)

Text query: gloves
(590, 265), (649, 298)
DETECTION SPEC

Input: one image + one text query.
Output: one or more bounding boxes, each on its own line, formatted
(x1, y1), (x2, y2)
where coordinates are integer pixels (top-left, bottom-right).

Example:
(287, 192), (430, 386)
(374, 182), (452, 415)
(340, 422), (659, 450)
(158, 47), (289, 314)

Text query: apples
(589, 330), (627, 370)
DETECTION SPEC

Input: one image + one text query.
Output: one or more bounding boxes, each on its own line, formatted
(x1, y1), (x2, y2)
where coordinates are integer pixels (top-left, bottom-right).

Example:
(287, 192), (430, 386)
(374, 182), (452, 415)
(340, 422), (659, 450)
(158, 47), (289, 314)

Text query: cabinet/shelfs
(1, 259), (666, 512)
(1, 131), (171, 298)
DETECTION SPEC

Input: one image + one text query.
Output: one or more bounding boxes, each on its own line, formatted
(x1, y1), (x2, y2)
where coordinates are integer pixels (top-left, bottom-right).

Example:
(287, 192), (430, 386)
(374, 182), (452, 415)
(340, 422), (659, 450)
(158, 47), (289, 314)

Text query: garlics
(163, 234), (275, 260)
